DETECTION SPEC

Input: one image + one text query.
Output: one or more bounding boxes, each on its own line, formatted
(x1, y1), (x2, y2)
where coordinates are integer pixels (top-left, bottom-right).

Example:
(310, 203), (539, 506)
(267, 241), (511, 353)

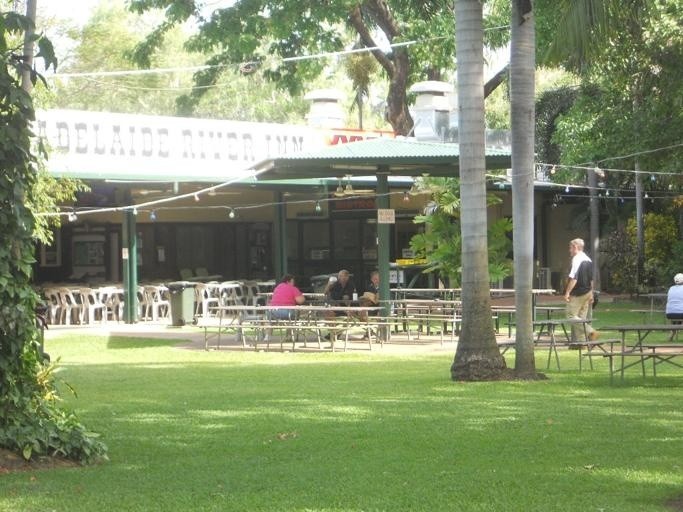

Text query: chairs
(34, 280), (277, 325)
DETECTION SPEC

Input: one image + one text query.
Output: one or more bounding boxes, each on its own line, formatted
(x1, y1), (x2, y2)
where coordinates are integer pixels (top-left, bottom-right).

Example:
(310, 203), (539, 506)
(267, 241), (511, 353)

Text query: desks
(390, 288), (557, 333)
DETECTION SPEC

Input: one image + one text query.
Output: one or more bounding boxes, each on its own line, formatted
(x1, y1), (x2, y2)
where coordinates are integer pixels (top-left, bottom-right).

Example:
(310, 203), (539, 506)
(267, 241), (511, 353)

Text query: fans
(334, 174), (345, 197)
(316, 174), (376, 194)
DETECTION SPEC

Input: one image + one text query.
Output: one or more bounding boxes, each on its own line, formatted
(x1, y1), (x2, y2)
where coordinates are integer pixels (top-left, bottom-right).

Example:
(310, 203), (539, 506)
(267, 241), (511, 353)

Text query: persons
(267, 273), (305, 341)
(665, 273), (683, 341)
(324, 269), (359, 340)
(359, 271), (392, 340)
(564, 238), (599, 350)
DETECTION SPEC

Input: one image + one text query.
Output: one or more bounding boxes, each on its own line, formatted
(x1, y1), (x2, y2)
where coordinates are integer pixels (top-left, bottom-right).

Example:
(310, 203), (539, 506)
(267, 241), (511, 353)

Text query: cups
(352, 293), (359, 301)
(329, 276), (338, 282)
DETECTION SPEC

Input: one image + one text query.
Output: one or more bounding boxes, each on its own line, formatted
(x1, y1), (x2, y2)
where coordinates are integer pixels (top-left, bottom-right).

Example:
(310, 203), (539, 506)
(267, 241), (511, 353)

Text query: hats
(674, 273), (683, 284)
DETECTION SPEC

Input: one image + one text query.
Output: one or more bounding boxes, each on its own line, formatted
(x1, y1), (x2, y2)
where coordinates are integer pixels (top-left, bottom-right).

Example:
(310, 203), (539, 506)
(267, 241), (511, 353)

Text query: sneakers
(588, 331), (600, 350)
(568, 344), (586, 350)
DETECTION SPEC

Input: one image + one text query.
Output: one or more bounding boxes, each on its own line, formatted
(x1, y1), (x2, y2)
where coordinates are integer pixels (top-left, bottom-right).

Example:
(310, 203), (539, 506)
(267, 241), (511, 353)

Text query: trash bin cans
(310, 272), (354, 320)
(165, 280), (198, 326)
